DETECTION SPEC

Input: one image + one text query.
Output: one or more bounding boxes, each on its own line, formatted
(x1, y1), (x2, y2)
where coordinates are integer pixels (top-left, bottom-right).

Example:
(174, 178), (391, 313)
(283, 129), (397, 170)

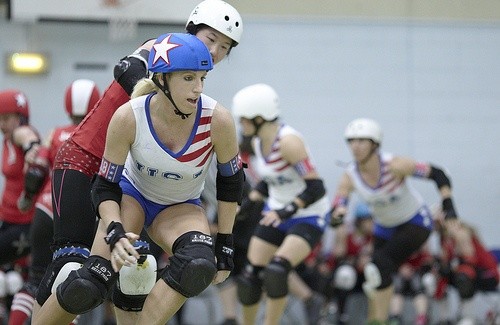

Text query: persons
(36, 33), (245, 325)
(215, 83), (500, 325)
(31, 1), (243, 325)
(0, 78), (102, 325)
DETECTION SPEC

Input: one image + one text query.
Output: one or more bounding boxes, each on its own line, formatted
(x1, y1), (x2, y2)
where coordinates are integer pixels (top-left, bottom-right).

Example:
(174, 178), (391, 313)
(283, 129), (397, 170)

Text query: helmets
(343, 118), (382, 147)
(0, 88), (30, 116)
(65, 79), (101, 116)
(231, 83), (280, 122)
(146, 33), (213, 73)
(354, 200), (374, 219)
(184, 0), (243, 48)
